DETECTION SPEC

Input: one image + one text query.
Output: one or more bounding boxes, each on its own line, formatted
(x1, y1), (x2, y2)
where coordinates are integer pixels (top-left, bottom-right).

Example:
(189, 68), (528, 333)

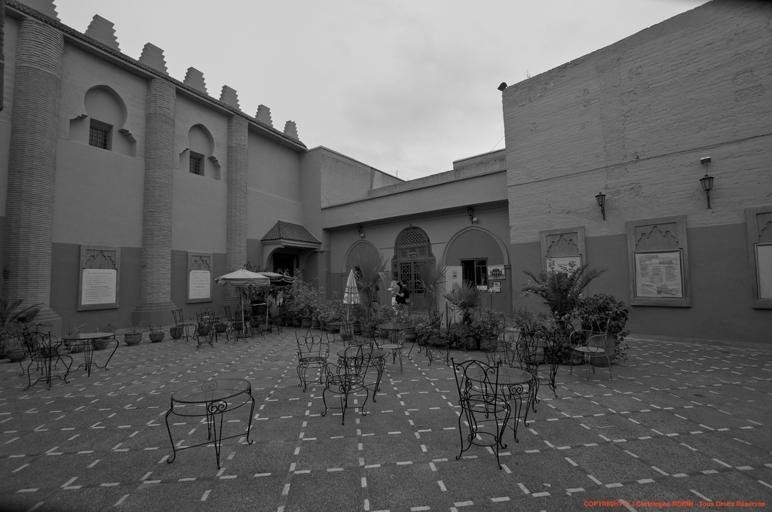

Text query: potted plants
(579, 293), (633, 367)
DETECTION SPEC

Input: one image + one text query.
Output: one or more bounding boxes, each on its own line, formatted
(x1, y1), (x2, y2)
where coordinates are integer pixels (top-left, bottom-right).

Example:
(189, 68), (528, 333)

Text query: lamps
(593, 190), (610, 223)
(699, 170), (719, 210)
(465, 206), (476, 220)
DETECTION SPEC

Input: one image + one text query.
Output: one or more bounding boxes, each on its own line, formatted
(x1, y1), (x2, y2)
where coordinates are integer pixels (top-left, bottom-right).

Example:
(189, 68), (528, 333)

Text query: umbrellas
(255, 268), (297, 328)
(342, 267), (360, 321)
(214, 267), (271, 333)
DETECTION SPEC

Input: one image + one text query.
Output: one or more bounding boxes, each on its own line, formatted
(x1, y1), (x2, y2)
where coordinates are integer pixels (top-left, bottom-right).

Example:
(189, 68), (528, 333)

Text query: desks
(64, 333), (119, 375)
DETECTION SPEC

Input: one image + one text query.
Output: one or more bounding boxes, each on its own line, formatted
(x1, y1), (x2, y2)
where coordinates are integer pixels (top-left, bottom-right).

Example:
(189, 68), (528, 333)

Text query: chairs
(12, 322), (58, 377)
(22, 330), (73, 390)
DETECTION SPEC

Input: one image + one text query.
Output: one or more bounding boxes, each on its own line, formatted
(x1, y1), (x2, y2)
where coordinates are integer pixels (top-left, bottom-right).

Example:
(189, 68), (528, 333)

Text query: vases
(7, 316), (262, 361)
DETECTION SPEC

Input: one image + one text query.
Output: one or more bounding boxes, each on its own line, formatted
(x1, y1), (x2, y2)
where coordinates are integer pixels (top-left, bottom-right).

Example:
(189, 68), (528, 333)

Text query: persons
(371, 279), (413, 322)
